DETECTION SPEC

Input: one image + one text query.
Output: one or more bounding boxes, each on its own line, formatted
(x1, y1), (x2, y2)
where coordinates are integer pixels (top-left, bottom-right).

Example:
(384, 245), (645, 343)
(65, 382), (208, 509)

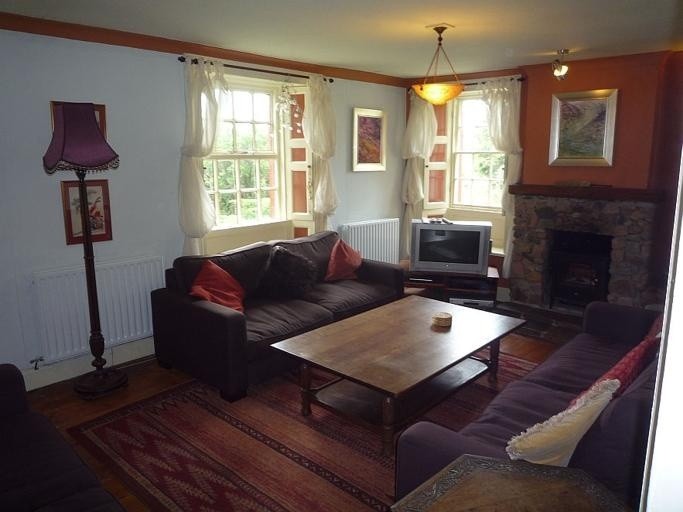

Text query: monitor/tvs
(409, 218), (492, 275)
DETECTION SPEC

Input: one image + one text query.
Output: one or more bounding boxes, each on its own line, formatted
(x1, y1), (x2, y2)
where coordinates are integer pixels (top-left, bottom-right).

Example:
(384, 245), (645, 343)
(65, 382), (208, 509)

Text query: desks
(392, 452), (643, 512)
(402, 261), (498, 385)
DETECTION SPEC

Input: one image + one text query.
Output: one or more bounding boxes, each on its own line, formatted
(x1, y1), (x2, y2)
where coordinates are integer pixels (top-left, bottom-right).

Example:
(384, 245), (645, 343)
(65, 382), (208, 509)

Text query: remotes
(409, 277), (433, 282)
(437, 217), (442, 223)
(422, 217), (429, 224)
(430, 217), (436, 224)
(442, 218), (453, 224)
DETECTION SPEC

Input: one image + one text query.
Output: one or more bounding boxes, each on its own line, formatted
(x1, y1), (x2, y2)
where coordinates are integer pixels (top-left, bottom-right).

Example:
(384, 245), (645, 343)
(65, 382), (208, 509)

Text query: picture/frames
(349, 106), (387, 172)
(47, 101), (107, 144)
(58, 177), (114, 247)
(546, 88), (615, 169)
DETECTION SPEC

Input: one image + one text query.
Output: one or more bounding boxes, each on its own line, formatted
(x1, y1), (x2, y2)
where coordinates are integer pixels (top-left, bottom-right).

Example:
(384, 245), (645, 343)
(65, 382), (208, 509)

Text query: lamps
(42, 101), (127, 398)
(408, 26), (466, 105)
(550, 47), (570, 81)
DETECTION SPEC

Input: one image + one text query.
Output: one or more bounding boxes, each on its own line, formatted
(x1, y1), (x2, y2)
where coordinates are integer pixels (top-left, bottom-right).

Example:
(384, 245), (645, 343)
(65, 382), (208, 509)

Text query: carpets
(68, 344), (538, 512)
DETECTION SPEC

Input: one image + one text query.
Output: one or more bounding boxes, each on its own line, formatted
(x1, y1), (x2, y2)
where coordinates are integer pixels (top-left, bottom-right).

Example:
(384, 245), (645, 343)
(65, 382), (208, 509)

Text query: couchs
(146, 228), (404, 403)
(393, 289), (667, 506)
(1, 359), (129, 512)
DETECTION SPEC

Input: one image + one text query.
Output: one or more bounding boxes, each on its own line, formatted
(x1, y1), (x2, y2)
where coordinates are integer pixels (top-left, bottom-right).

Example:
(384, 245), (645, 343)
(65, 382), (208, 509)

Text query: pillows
(324, 235), (365, 287)
(254, 240), (322, 301)
(188, 258), (248, 318)
(507, 315), (665, 464)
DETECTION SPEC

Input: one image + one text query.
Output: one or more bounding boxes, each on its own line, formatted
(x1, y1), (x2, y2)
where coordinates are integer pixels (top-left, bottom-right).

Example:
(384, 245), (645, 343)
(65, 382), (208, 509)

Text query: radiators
(338, 217), (400, 266)
(1, 254), (166, 377)
